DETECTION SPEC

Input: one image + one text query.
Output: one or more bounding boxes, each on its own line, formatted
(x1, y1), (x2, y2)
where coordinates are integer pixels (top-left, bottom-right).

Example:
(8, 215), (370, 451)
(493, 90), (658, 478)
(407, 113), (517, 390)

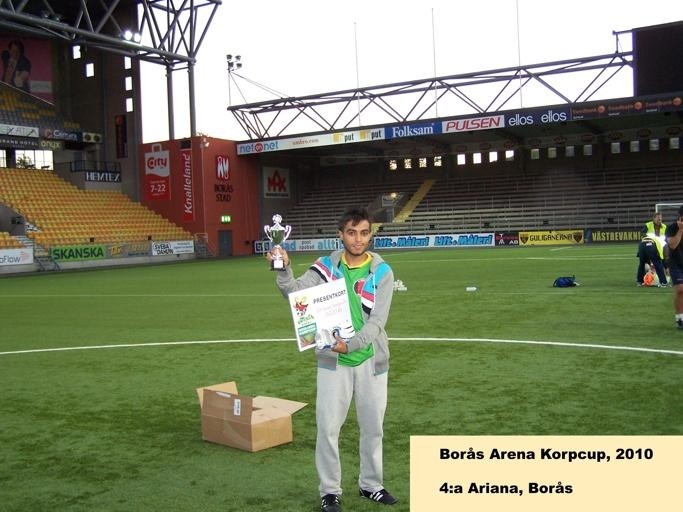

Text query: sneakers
(637, 282), (642, 287)
(358, 488), (398, 506)
(321, 494), (341, 512)
(676, 319), (682, 329)
(661, 283), (668, 288)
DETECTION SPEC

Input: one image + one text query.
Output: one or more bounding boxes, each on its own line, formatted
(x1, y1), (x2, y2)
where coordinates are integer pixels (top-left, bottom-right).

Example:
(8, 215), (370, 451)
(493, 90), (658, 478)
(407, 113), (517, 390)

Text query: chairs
(0, 167), (199, 248)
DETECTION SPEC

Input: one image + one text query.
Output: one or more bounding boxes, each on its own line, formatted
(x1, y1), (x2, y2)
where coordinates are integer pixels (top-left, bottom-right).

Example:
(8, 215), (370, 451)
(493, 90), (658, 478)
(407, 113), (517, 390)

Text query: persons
(666, 204), (683, 329)
(636, 236), (669, 288)
(640, 213), (669, 274)
(1, 40), (32, 94)
(266, 210), (398, 512)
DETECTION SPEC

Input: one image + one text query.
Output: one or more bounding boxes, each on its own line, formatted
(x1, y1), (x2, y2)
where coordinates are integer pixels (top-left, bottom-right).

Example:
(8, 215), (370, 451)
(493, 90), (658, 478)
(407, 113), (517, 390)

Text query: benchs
(280, 160), (682, 230)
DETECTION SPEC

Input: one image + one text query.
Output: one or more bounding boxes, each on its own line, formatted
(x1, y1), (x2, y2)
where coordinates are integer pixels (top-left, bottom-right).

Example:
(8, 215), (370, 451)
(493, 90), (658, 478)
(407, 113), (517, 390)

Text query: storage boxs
(191, 380), (308, 454)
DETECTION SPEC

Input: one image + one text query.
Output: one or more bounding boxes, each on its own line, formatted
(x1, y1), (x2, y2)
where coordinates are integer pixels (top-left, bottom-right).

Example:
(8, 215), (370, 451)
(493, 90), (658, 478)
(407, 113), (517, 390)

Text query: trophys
(264, 215), (292, 270)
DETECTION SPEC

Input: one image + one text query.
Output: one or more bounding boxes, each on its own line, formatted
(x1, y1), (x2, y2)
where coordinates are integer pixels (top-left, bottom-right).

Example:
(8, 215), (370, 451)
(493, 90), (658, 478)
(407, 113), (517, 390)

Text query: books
(287, 277), (356, 353)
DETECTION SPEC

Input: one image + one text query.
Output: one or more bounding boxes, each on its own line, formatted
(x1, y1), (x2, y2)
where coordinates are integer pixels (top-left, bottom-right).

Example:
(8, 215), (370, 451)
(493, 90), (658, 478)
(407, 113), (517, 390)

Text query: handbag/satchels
(553, 276), (576, 287)
